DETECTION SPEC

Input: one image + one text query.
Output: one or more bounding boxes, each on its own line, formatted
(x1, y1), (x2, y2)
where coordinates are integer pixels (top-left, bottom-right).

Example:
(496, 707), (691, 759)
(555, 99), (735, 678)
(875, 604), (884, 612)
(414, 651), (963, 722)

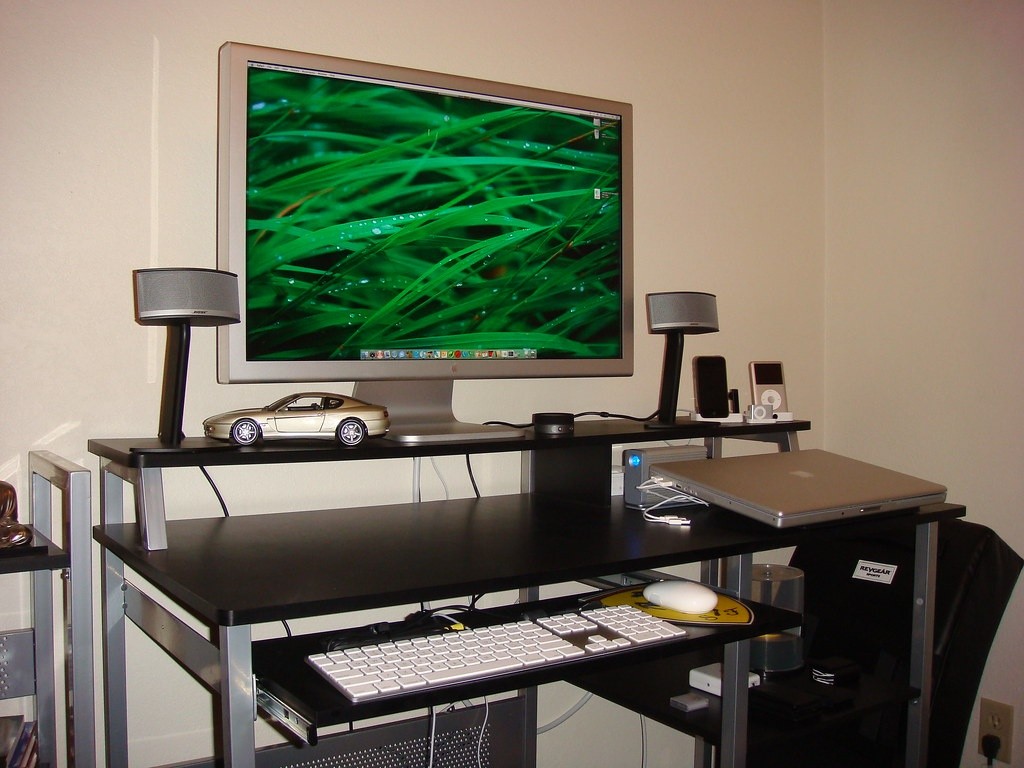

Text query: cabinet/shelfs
(0, 449), (93, 768)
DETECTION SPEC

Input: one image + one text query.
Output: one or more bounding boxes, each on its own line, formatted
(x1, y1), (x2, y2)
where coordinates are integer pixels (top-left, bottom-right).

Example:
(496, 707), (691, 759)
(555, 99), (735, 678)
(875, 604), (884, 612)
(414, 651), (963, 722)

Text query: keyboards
(308, 604), (688, 705)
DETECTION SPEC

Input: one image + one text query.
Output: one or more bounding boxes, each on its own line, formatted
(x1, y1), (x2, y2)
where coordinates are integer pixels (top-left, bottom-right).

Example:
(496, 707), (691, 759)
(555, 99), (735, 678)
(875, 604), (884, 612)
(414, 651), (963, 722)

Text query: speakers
(129, 267), (242, 454)
(641, 291), (721, 428)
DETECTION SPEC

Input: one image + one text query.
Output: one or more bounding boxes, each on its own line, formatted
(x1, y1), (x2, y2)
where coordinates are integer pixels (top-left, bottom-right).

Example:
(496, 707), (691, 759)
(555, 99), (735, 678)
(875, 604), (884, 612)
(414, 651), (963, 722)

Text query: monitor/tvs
(215, 42), (635, 444)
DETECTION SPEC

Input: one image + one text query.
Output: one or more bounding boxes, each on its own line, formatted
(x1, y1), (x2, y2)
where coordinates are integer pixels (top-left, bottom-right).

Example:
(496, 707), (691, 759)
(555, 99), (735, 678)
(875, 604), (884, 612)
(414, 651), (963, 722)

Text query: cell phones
(694, 355), (729, 419)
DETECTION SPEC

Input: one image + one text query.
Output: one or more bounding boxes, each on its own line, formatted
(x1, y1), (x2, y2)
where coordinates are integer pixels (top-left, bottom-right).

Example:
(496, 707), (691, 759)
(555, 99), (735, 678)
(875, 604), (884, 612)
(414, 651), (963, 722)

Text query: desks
(85, 494), (1016, 767)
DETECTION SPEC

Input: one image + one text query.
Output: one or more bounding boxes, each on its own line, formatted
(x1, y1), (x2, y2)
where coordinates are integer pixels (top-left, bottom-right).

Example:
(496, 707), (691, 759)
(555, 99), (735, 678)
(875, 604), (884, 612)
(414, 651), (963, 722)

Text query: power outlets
(977, 696), (1012, 764)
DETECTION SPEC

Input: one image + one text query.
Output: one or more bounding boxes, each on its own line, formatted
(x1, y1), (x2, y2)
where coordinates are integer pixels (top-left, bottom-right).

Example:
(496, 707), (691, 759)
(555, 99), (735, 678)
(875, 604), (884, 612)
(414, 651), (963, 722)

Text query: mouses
(643, 580), (718, 614)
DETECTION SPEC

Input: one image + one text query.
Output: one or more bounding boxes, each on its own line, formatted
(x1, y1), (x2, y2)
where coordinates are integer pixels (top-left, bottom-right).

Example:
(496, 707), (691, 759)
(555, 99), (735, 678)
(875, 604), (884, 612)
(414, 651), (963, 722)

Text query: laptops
(648, 447), (947, 528)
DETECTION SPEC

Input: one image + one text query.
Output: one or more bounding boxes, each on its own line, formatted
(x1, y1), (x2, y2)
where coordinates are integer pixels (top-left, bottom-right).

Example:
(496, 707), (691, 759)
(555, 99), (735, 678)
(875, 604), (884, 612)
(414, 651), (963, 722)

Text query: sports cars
(203, 392), (390, 447)
